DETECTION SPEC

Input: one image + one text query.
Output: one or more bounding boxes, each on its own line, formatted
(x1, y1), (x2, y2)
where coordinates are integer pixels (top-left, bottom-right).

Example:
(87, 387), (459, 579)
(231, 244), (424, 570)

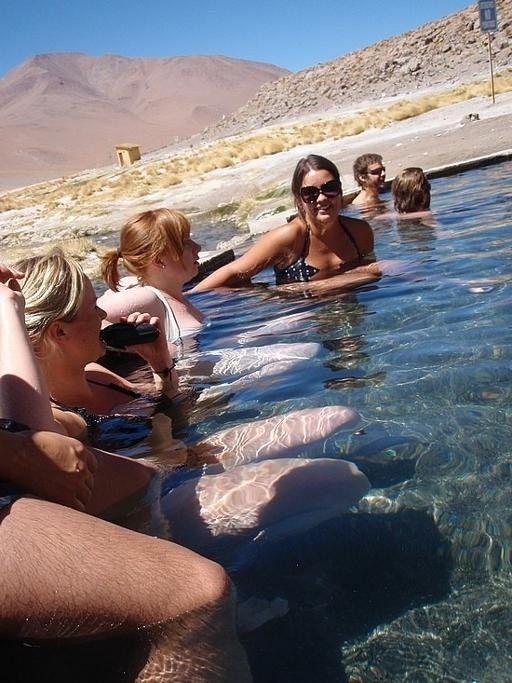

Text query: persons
(187, 154), (403, 294)
(0, 246), (371, 575)
(0, 419), (250, 683)
(374, 166), (432, 221)
(95, 206), (322, 404)
(350, 154), (387, 204)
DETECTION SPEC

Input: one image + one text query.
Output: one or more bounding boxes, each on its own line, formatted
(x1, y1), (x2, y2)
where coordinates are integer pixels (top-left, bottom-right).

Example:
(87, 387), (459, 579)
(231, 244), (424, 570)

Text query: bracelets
(151, 362), (175, 381)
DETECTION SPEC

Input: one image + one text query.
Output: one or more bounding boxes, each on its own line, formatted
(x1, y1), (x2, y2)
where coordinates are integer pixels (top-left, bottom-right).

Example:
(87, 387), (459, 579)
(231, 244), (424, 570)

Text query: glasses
(364, 166), (386, 175)
(298, 179), (342, 203)
